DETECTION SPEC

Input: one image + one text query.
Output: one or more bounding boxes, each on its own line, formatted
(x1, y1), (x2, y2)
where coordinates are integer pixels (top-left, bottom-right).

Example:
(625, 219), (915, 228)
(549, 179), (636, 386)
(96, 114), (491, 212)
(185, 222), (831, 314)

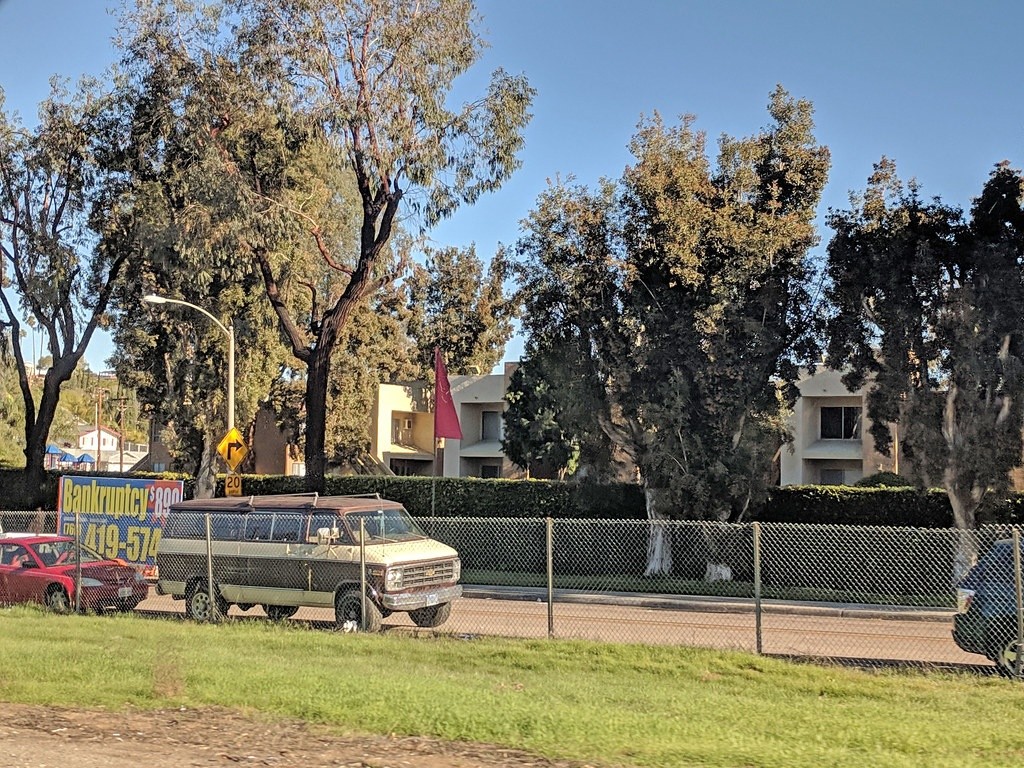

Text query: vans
(154, 491), (462, 635)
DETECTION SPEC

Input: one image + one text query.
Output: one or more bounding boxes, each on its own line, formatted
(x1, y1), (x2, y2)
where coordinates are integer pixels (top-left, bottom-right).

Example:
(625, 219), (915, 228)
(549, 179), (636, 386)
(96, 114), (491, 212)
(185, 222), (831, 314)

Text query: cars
(951, 539), (1024, 680)
(0, 534), (150, 615)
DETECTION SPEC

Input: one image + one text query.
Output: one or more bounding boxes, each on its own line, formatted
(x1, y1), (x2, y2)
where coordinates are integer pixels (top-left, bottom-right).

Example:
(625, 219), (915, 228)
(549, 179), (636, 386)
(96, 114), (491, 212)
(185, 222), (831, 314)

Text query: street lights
(143, 295), (236, 476)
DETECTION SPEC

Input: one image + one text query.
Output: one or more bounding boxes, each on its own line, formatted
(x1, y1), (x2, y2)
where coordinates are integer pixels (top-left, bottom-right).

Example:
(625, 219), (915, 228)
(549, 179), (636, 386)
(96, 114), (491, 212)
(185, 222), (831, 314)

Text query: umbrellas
(47, 444), (96, 470)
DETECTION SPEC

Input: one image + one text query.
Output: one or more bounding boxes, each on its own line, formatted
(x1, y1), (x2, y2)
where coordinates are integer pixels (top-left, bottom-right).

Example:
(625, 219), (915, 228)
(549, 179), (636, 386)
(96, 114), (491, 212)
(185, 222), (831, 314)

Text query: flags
(433, 347), (463, 441)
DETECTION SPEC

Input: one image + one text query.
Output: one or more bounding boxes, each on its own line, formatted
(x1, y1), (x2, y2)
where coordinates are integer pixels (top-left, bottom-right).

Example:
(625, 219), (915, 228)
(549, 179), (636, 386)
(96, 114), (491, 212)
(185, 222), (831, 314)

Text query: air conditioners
(403, 419), (412, 430)
